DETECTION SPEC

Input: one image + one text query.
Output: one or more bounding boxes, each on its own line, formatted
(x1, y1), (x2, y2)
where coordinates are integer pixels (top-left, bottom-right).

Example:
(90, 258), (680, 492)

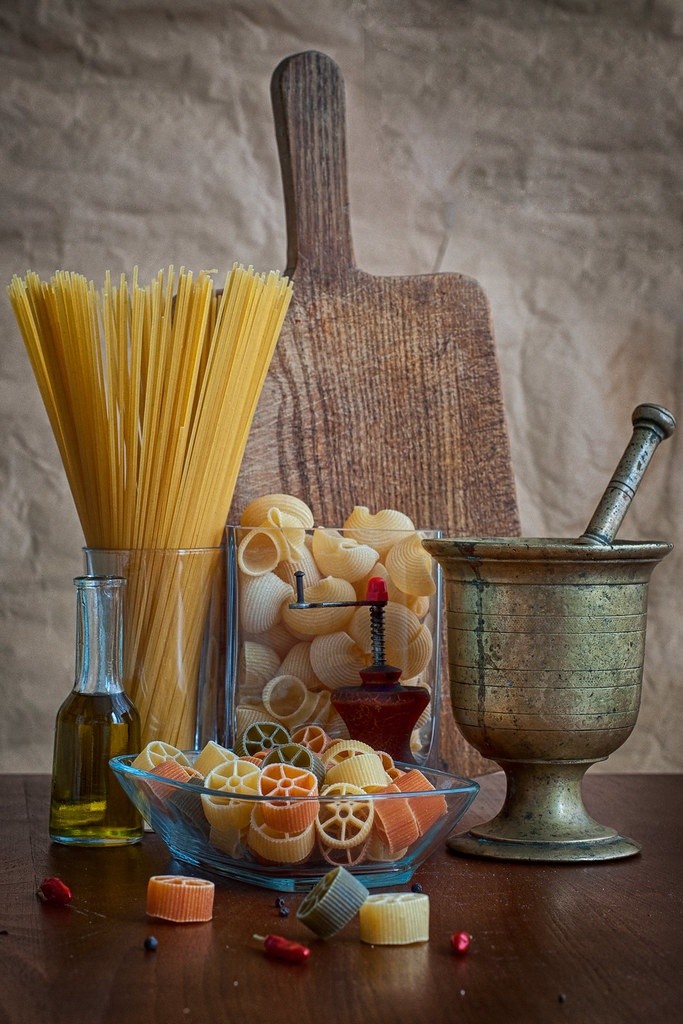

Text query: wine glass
(421, 536), (672, 861)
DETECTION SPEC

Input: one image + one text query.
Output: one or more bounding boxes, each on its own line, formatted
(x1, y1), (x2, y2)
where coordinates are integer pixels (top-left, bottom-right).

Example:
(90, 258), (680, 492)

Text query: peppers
(452, 932), (470, 952)
(251, 934), (311, 961)
(41, 877), (72, 903)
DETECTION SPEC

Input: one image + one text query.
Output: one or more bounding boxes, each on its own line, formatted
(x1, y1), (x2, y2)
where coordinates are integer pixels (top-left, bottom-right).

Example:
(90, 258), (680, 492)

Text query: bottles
(49, 577), (142, 847)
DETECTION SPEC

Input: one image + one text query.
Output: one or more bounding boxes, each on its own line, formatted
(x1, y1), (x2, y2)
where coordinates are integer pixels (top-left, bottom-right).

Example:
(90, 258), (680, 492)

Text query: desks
(0, 771), (683, 1024)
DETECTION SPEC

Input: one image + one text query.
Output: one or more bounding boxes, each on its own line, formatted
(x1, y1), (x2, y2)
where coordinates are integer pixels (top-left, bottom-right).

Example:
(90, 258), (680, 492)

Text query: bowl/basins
(109, 751), (480, 885)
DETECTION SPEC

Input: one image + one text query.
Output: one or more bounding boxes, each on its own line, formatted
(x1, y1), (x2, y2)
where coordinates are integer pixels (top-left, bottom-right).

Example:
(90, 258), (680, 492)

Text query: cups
(224, 526), (442, 773)
(82, 547), (223, 831)
